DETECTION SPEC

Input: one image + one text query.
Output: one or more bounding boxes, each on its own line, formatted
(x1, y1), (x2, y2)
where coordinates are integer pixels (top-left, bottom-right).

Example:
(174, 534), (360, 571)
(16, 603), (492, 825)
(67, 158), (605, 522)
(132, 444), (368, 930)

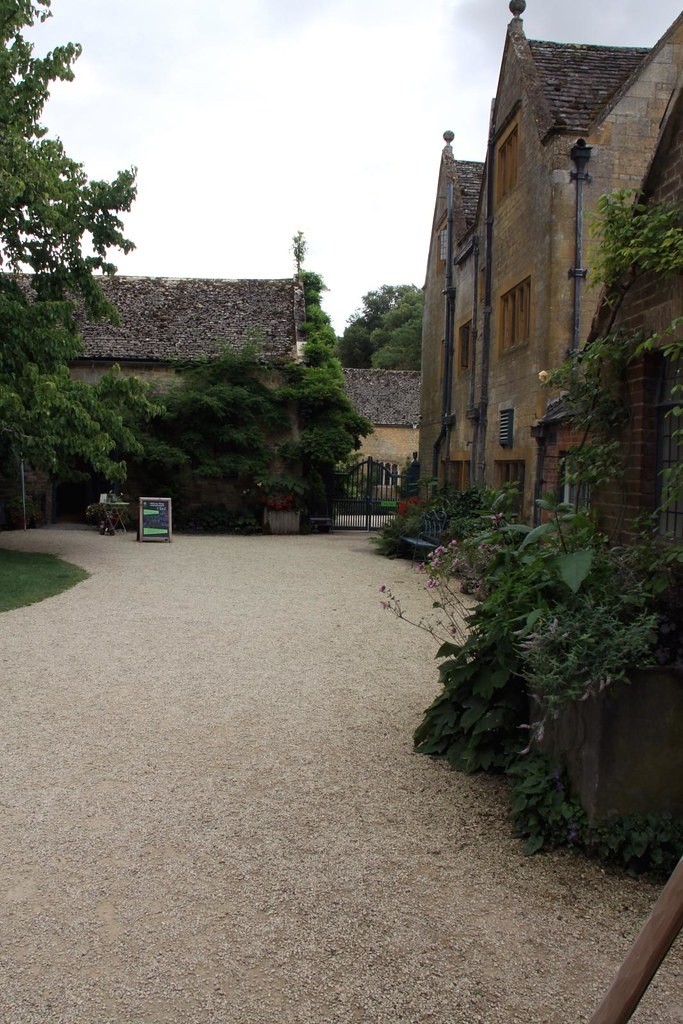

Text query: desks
(98, 502), (130, 536)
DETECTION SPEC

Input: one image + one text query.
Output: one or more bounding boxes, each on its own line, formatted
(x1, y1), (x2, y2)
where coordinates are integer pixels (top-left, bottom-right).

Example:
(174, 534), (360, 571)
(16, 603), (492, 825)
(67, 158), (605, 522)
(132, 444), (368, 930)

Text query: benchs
(399, 509), (447, 569)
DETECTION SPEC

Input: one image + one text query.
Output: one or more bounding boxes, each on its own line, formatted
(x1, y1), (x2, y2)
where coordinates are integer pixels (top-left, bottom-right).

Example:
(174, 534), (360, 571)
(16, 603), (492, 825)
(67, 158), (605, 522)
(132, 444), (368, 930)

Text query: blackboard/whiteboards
(139, 497), (172, 541)
